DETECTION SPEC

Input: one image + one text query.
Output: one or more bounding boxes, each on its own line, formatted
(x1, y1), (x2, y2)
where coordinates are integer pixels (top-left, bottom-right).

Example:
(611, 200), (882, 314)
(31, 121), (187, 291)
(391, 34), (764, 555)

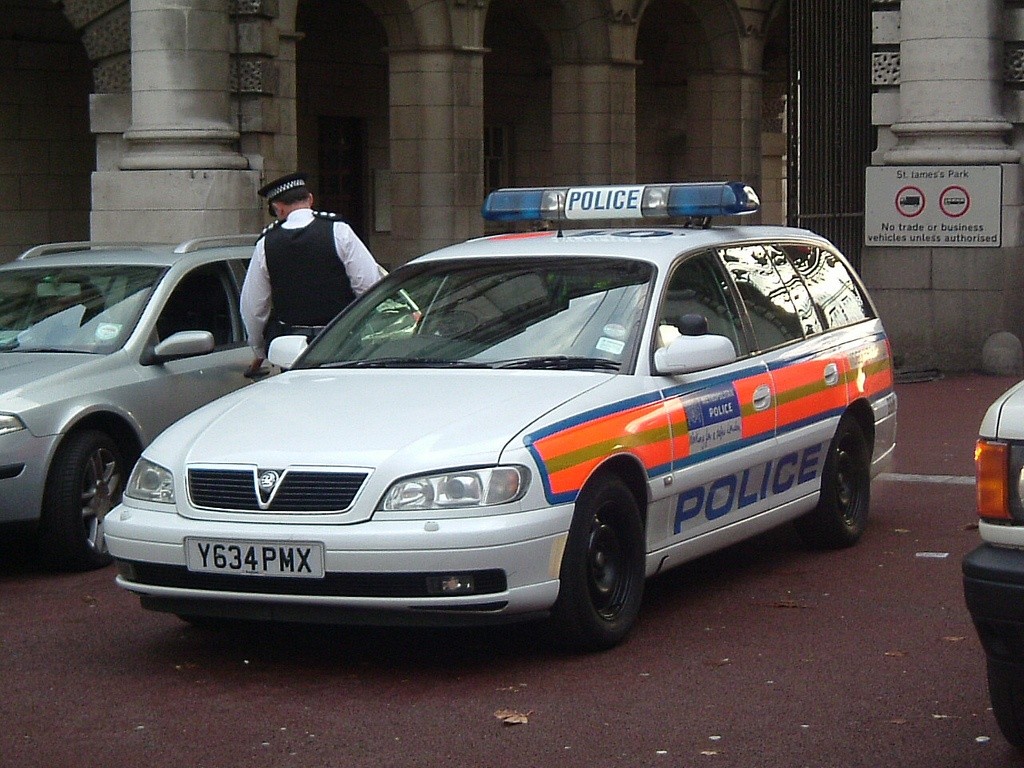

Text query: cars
(100, 181), (900, 651)
(0, 233), (441, 570)
(959, 377), (1023, 750)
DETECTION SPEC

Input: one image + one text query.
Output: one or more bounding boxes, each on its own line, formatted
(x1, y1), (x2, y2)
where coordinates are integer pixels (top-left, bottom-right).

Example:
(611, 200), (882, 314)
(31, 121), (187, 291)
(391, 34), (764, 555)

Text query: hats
(257, 172), (312, 218)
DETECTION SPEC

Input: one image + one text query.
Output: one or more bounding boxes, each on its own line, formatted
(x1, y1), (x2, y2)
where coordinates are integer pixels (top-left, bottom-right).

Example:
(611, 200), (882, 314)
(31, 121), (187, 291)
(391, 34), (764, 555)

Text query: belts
(291, 325), (326, 336)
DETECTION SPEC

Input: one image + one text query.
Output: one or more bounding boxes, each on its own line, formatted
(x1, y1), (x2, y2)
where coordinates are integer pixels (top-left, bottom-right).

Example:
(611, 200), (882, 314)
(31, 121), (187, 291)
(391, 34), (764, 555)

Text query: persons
(240, 170), (381, 373)
